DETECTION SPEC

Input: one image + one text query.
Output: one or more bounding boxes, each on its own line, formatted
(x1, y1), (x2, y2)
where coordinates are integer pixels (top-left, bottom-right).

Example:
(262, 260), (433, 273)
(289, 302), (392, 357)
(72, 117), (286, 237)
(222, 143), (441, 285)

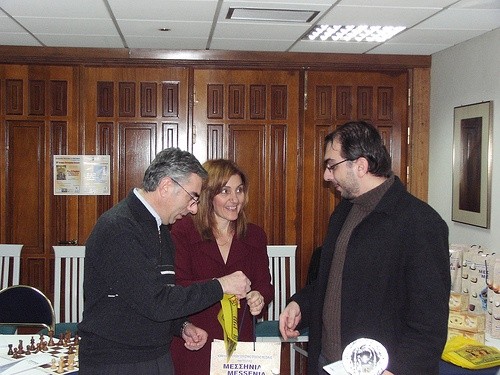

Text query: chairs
(0, 284), (56, 337)
(257, 245), (309, 375)
(0, 245), (24, 334)
(37, 245), (85, 338)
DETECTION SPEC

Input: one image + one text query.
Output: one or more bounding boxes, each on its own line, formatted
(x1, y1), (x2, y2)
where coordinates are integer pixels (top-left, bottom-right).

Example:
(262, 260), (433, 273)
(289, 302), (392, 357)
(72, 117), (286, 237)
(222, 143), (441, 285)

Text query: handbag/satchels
(210, 300), (281, 375)
(440, 244), (500, 369)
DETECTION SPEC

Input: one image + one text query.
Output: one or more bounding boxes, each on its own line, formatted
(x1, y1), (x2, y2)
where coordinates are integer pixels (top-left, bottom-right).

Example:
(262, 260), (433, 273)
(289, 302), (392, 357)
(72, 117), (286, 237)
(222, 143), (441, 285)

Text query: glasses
(323, 154), (369, 173)
(169, 176), (200, 208)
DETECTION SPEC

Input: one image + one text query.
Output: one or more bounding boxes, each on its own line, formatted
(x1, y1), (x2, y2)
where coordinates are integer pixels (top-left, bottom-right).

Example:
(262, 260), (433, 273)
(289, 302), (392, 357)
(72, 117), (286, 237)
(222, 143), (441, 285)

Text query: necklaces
(216, 228), (232, 247)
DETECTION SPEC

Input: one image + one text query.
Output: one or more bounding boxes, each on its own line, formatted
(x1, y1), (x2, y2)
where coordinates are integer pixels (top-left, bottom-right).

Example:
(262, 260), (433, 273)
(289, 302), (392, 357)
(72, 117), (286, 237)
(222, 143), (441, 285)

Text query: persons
(171, 159), (272, 375)
(77, 147), (251, 375)
(278, 120), (450, 375)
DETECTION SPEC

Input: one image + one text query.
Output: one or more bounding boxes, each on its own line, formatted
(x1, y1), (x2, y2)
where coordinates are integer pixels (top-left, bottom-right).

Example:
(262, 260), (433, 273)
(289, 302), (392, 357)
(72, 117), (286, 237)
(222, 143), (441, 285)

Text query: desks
(0, 334), (82, 375)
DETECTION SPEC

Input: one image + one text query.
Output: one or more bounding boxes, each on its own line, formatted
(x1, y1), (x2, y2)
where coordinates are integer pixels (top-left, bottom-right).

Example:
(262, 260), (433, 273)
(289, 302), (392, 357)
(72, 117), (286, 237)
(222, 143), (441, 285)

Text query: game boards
(0, 334), (80, 374)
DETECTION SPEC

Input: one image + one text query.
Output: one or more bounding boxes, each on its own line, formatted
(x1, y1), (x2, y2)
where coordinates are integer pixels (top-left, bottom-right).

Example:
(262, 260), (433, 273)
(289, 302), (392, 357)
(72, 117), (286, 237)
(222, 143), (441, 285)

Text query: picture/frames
(451, 101), (493, 230)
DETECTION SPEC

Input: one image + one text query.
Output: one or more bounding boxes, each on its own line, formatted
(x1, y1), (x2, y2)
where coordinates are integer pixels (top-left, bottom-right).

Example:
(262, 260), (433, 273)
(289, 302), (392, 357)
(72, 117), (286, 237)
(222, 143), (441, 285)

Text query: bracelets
(180, 323), (191, 336)
